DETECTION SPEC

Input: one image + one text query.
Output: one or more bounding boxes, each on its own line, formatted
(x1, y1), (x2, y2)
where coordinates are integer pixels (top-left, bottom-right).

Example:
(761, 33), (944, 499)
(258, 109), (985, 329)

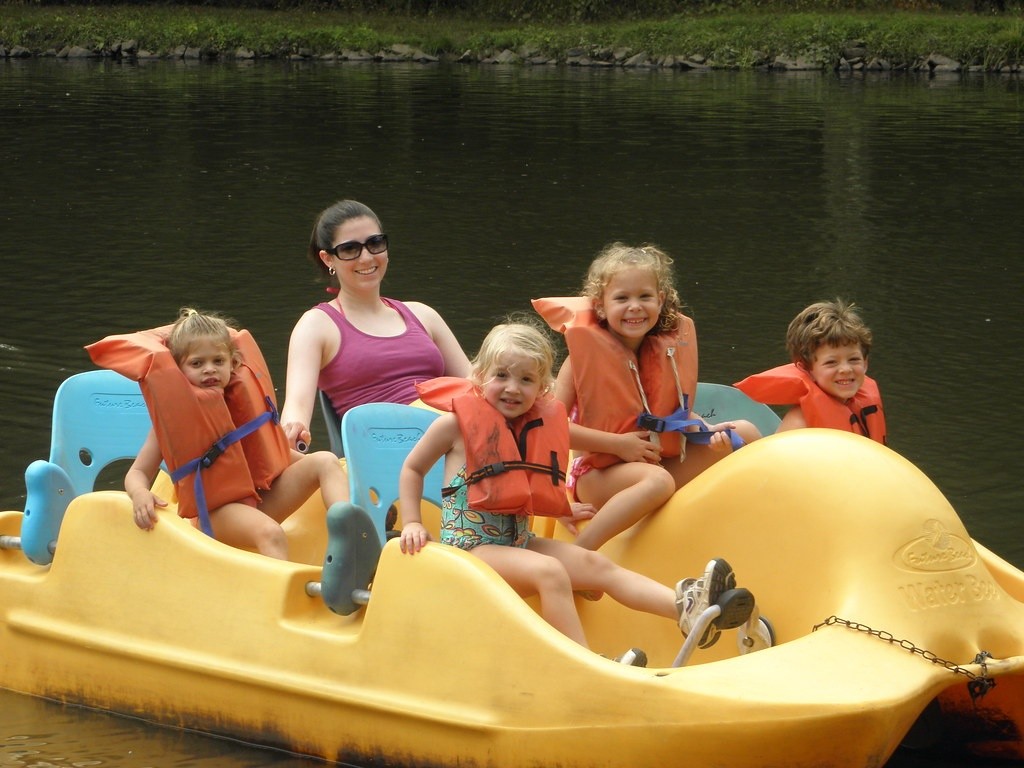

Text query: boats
(0, 369), (1024, 768)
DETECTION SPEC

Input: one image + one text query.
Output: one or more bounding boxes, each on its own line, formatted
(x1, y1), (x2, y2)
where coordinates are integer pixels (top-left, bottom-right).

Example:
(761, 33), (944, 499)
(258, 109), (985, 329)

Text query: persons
(397, 318), (736, 669)
(531, 238), (762, 602)
(278, 200), (477, 451)
(734, 298), (888, 445)
(82, 304), (350, 560)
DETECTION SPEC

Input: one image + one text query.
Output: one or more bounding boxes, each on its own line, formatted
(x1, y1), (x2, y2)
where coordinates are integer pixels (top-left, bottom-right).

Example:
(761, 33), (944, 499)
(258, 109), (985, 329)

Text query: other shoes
(385, 505), (397, 531)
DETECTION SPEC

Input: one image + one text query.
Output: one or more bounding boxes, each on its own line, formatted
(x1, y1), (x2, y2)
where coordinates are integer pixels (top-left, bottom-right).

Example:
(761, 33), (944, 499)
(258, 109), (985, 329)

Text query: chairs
(319, 388), (345, 459)
(20, 370), (174, 566)
(342, 401), (446, 550)
(691, 381), (783, 437)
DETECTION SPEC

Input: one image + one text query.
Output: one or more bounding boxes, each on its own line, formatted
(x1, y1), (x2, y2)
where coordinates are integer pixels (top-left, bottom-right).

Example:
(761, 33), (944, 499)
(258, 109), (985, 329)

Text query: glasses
(328, 233), (389, 261)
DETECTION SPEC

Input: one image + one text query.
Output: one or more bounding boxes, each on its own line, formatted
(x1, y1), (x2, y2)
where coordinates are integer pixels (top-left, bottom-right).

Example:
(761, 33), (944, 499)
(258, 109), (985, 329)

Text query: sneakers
(598, 648), (647, 667)
(675, 558), (737, 649)
